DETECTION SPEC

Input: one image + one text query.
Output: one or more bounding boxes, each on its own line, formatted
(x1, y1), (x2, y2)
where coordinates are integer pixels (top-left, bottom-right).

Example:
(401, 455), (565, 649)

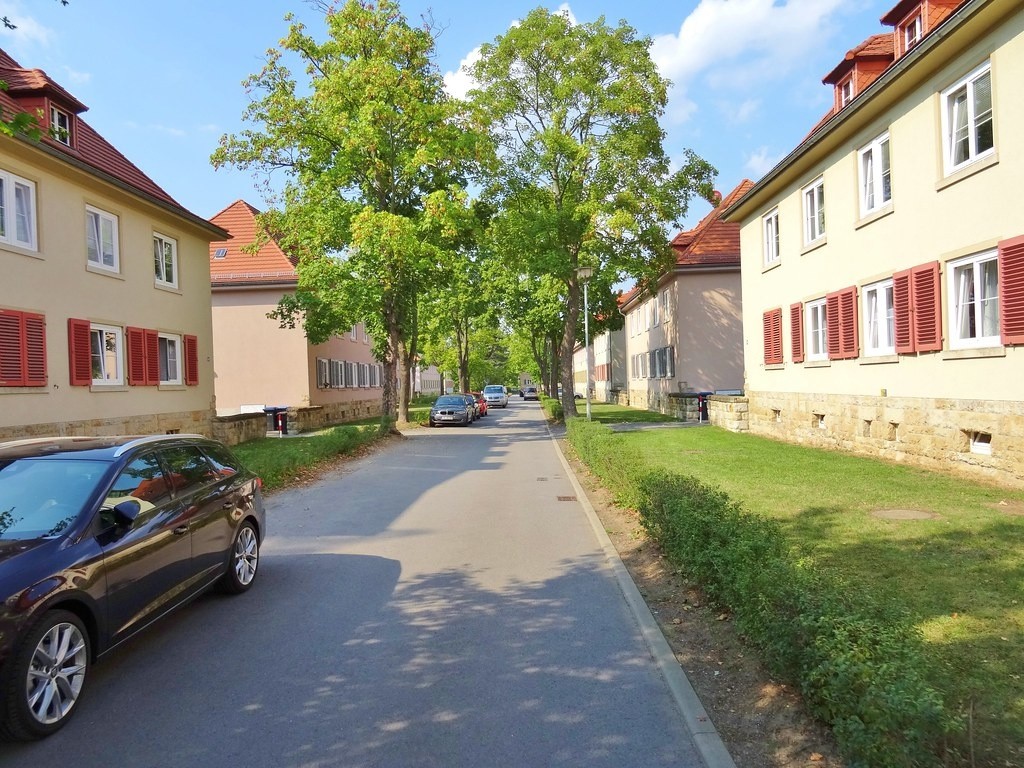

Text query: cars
(549, 388), (583, 399)
(461, 392), (488, 419)
(523, 386), (539, 401)
(0, 435), (265, 741)
(429, 394), (472, 427)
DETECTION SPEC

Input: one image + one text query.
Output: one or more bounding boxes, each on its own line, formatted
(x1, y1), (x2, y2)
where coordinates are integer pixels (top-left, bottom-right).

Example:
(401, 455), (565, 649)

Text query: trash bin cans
(697, 391), (714, 420)
(262, 407), (288, 435)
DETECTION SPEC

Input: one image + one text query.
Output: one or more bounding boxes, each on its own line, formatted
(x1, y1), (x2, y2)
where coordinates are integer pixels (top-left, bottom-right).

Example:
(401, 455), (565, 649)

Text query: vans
(480, 385), (509, 408)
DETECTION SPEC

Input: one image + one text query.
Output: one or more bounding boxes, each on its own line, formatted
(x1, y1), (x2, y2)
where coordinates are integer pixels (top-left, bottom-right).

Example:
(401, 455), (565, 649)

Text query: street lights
(572, 266), (594, 422)
